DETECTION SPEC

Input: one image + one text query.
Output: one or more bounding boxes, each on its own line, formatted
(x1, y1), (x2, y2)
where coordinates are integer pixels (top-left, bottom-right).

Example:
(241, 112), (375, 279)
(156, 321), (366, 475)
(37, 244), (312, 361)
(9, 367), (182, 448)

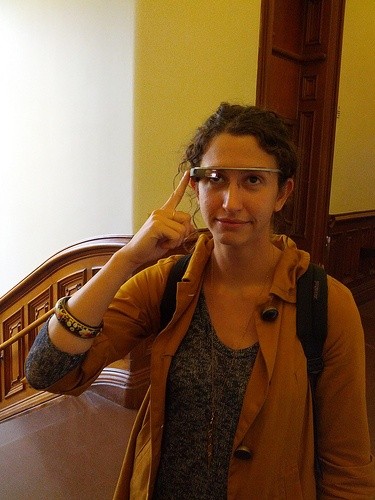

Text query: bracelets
(53, 296), (105, 339)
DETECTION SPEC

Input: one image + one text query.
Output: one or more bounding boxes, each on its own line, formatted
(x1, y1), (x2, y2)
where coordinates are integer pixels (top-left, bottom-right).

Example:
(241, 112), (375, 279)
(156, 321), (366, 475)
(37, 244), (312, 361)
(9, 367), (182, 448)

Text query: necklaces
(196, 241), (275, 470)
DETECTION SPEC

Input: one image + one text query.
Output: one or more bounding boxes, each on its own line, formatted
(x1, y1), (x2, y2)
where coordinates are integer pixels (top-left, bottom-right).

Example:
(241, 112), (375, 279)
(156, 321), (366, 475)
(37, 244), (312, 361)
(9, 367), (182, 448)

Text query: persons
(23, 99), (375, 499)
